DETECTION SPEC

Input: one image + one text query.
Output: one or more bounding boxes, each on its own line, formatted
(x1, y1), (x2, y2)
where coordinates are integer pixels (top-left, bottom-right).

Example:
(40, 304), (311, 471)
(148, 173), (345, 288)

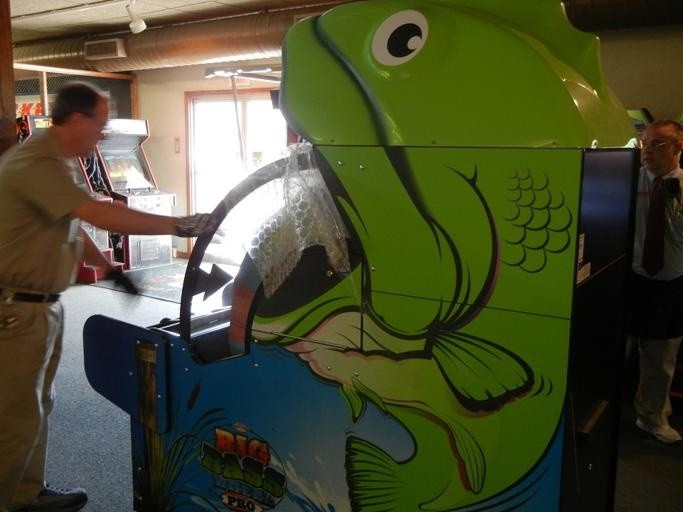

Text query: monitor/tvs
(101, 150), (155, 191)
(71, 156), (85, 187)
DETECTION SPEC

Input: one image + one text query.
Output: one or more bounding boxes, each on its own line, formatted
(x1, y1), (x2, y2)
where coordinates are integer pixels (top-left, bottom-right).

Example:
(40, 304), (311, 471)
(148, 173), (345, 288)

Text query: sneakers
(25, 484), (89, 511)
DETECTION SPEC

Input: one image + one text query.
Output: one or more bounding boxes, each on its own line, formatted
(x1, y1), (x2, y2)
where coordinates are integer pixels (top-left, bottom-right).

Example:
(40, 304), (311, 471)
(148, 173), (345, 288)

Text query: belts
(12, 292), (62, 303)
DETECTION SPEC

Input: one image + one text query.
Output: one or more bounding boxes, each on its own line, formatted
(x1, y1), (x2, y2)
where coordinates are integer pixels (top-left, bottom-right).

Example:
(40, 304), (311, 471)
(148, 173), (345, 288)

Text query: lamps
(124, 1), (146, 35)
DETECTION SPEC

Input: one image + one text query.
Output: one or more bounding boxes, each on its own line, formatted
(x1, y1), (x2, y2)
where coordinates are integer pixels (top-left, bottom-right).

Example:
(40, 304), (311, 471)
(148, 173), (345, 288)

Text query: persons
(0, 83), (222, 511)
(631, 121), (681, 444)
